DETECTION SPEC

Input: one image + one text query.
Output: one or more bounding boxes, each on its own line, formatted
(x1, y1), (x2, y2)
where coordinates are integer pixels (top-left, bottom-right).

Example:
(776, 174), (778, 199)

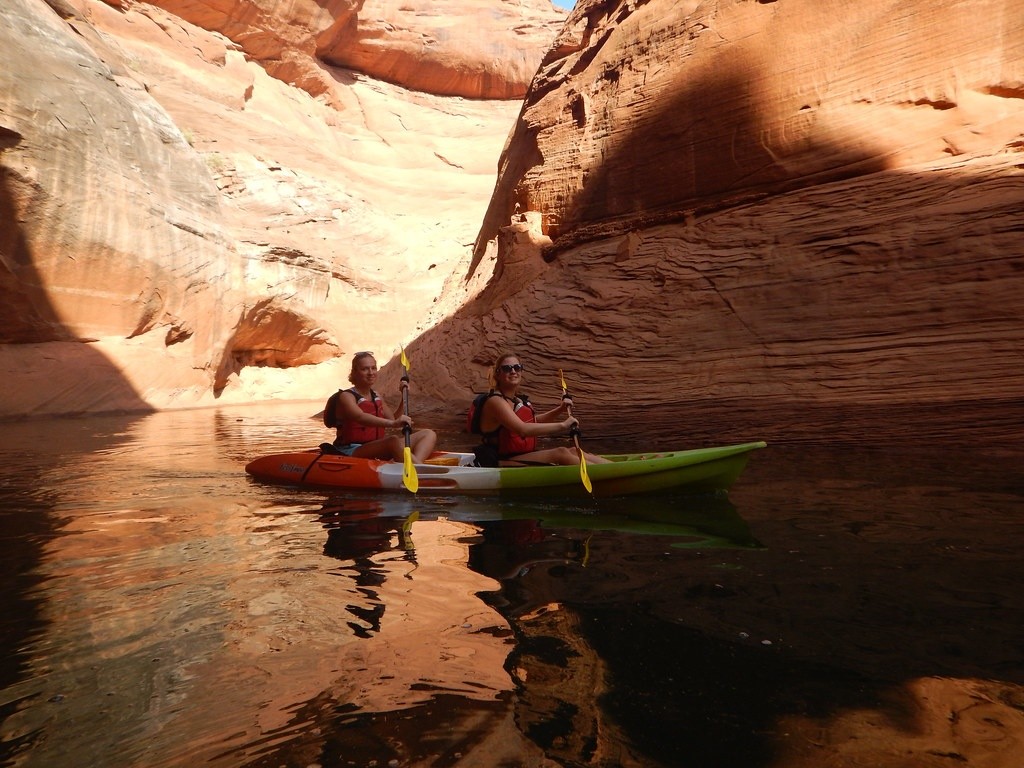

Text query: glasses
(354, 351), (374, 359)
(495, 363), (523, 372)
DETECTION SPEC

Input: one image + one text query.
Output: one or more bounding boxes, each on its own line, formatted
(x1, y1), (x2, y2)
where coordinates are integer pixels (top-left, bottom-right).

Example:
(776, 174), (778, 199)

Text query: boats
(242, 436), (772, 499)
(302, 483), (774, 553)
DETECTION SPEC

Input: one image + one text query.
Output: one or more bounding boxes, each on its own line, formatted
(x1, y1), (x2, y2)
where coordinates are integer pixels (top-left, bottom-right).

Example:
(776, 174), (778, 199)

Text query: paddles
(559, 368), (593, 494)
(398, 341), (420, 494)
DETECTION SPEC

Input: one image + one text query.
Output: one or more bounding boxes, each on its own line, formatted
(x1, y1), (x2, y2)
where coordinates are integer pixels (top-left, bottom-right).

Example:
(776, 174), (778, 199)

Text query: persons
(468, 353), (614, 464)
(324, 351), (437, 464)
